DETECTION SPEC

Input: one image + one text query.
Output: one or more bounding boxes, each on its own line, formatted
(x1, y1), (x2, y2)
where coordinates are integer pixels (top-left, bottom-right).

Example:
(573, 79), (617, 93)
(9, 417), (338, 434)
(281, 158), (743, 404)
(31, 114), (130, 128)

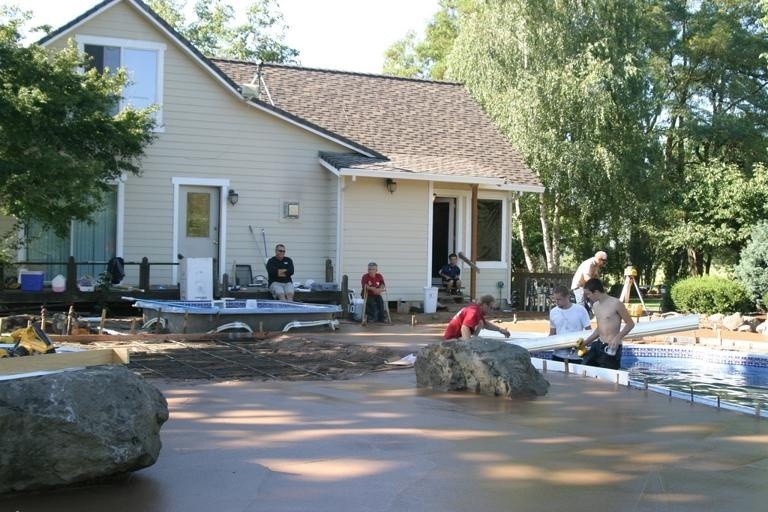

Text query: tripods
(250, 75), (276, 106)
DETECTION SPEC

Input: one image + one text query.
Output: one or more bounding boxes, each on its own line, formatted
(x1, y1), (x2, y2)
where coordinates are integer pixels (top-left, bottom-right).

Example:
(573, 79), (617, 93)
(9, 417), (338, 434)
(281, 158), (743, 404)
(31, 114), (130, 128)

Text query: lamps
(229, 189), (238, 206)
(386, 179), (397, 195)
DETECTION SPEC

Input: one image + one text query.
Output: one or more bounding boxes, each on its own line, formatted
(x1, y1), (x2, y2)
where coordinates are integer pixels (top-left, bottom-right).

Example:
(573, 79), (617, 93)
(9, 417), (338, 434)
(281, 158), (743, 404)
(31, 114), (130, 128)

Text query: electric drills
(566, 338), (587, 361)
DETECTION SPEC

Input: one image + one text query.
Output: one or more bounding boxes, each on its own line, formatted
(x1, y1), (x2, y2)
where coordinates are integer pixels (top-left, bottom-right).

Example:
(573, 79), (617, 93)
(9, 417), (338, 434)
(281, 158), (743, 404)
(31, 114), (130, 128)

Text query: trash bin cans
(423, 287), (438, 313)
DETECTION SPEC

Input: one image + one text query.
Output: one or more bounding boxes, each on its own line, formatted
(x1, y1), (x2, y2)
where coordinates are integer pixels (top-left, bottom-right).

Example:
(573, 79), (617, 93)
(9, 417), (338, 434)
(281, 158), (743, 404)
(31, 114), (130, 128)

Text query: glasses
(279, 249), (285, 252)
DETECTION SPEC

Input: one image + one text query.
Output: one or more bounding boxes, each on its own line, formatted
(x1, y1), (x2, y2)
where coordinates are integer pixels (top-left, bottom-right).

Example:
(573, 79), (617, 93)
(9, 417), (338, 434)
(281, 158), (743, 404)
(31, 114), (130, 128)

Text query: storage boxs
(21, 270), (44, 291)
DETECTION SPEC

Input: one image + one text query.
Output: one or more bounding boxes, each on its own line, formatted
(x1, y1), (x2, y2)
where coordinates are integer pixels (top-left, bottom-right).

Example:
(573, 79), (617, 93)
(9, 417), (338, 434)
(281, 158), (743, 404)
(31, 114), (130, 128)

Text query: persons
(265, 243), (296, 303)
(571, 250), (609, 316)
(358, 262), (387, 326)
(438, 254), (464, 296)
(548, 285), (592, 336)
(441, 294), (511, 341)
(574, 279), (636, 370)
